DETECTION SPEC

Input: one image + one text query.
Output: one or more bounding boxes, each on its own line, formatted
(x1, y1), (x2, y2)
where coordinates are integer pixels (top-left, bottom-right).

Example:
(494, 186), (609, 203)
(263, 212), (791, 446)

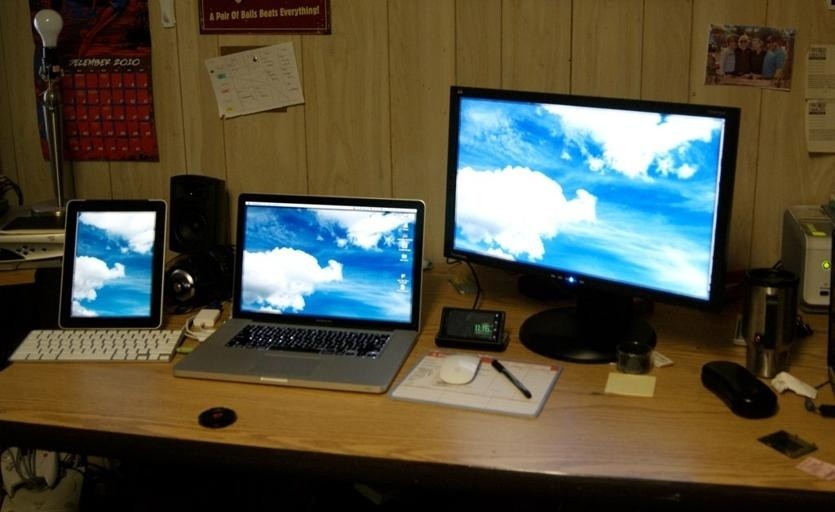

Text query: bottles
(745, 268), (800, 346)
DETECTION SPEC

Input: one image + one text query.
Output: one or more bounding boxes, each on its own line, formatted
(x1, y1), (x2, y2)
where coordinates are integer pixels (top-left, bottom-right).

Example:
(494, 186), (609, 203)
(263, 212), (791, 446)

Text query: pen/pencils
(491, 359), (531, 399)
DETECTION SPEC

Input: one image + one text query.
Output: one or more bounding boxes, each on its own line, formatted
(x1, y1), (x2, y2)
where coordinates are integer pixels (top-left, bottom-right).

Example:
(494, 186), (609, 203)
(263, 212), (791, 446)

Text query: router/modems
(782, 205), (835, 314)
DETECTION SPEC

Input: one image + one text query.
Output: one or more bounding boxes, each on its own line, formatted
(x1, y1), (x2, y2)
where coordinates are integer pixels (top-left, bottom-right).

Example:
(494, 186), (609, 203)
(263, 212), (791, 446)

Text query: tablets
(58, 199), (167, 329)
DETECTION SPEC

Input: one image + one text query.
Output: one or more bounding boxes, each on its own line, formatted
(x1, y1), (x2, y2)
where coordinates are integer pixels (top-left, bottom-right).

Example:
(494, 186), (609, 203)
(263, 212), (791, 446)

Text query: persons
(717, 33), (787, 79)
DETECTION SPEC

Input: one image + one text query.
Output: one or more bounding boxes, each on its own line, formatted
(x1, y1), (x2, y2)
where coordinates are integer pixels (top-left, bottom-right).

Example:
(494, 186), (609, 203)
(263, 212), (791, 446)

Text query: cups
(747, 340), (789, 379)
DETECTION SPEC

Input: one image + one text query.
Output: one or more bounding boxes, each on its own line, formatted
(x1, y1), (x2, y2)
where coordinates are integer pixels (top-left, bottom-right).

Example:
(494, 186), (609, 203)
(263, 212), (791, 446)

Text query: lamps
(30, 4), (73, 220)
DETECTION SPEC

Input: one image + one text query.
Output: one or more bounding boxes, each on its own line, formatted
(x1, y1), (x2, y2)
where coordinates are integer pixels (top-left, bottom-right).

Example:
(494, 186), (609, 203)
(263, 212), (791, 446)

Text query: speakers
(169, 175), (225, 308)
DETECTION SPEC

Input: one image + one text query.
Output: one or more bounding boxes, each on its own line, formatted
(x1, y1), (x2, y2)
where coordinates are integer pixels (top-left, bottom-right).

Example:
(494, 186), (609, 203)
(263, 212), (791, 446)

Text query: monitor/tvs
(443, 84), (741, 364)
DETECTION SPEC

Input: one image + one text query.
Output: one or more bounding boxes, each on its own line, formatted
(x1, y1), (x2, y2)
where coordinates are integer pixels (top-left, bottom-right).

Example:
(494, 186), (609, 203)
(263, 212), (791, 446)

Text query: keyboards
(8, 329), (183, 362)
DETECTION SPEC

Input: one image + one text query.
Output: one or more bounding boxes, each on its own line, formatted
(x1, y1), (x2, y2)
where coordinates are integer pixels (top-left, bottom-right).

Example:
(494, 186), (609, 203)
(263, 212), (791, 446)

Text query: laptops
(173, 193), (426, 394)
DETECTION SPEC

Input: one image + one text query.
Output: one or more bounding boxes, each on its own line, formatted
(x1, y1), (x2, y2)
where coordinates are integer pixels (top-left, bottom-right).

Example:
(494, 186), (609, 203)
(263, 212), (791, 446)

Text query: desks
(0, 261), (835, 511)
